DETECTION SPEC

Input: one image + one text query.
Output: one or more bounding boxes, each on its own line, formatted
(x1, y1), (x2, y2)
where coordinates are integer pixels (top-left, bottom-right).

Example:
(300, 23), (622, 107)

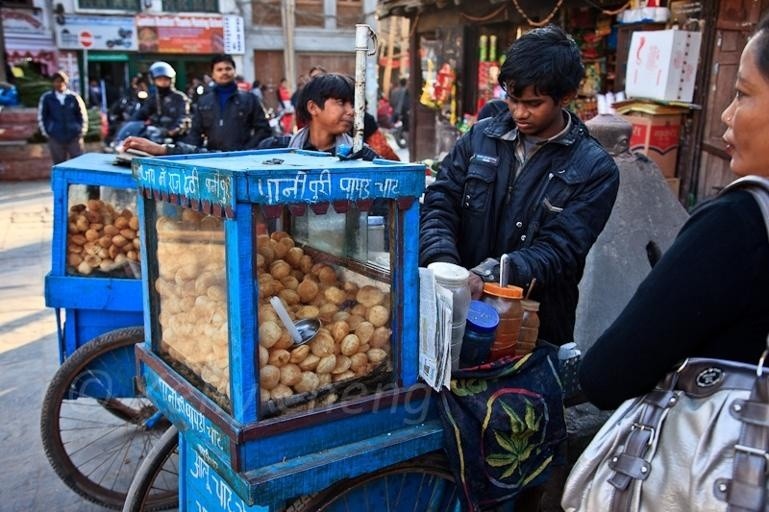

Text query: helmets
(147, 61), (176, 94)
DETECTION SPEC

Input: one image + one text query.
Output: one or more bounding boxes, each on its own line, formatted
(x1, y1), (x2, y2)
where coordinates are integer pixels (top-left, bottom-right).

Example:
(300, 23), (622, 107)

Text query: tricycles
(39, 148), (386, 511)
(105, 144), (584, 512)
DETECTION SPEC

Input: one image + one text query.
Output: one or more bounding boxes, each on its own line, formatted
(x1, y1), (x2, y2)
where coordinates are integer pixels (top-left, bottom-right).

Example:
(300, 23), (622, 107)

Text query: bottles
(428, 260), (471, 370)
(457, 298), (500, 369)
(514, 299), (543, 353)
(483, 282), (524, 362)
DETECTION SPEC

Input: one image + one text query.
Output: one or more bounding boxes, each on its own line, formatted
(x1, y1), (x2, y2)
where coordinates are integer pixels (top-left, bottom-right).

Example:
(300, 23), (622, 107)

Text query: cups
(575, 90), (630, 119)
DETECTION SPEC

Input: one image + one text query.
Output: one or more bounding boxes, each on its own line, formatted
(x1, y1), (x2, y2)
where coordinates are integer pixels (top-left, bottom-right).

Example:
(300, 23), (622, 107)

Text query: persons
(109, 61), (214, 142)
(577, 14), (769, 412)
(37, 71), (89, 165)
(183, 56), (271, 151)
(418, 25), (620, 348)
(123, 72), (390, 253)
(276, 65), (327, 134)
(236, 76), (264, 99)
(378, 78), (410, 129)
(91, 81), (102, 107)
(364, 100), (399, 160)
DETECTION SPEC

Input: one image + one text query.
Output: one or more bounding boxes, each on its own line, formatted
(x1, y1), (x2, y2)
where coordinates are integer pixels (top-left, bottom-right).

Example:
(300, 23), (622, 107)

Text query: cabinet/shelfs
(45, 152), (143, 312)
(132, 147), (426, 472)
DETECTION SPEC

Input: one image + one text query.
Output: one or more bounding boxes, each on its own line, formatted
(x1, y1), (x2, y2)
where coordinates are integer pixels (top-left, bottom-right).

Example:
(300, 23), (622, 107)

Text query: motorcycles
(102, 120), (176, 144)
(118, 90), (141, 119)
(391, 114), (407, 149)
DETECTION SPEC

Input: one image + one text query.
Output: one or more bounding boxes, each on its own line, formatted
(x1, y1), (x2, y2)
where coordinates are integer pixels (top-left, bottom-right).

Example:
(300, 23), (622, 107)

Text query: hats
(52, 71), (69, 82)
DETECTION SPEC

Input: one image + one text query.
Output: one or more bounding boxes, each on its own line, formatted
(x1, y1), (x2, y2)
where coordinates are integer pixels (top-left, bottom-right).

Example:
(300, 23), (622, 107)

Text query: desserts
(155, 208), (393, 404)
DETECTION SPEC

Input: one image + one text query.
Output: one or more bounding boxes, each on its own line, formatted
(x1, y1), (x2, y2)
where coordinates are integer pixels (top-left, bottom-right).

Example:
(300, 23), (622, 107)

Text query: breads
(67, 197), (142, 277)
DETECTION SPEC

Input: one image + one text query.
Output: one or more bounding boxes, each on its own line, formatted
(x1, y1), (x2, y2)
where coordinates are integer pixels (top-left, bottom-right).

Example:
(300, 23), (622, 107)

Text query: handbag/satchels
(561, 354), (769, 512)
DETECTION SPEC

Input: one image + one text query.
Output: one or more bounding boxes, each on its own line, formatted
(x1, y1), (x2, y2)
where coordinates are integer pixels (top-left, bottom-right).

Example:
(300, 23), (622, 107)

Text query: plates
(291, 317), (321, 345)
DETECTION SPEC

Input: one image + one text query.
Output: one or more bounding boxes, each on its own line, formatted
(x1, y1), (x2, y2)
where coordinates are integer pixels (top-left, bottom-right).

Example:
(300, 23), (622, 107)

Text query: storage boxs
(621, 114), (682, 177)
